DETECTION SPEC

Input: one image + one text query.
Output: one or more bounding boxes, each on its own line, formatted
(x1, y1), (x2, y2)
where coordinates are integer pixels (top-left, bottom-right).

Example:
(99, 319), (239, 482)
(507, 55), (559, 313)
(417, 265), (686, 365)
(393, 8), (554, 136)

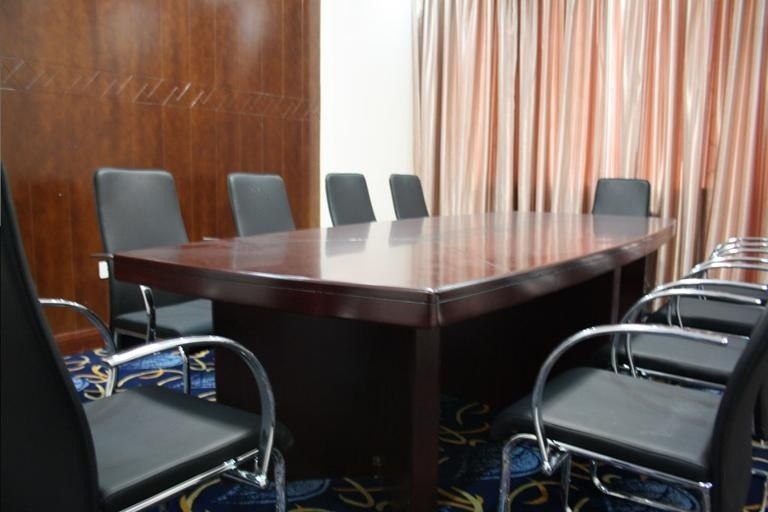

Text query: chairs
(0, 156), (768, 511)
